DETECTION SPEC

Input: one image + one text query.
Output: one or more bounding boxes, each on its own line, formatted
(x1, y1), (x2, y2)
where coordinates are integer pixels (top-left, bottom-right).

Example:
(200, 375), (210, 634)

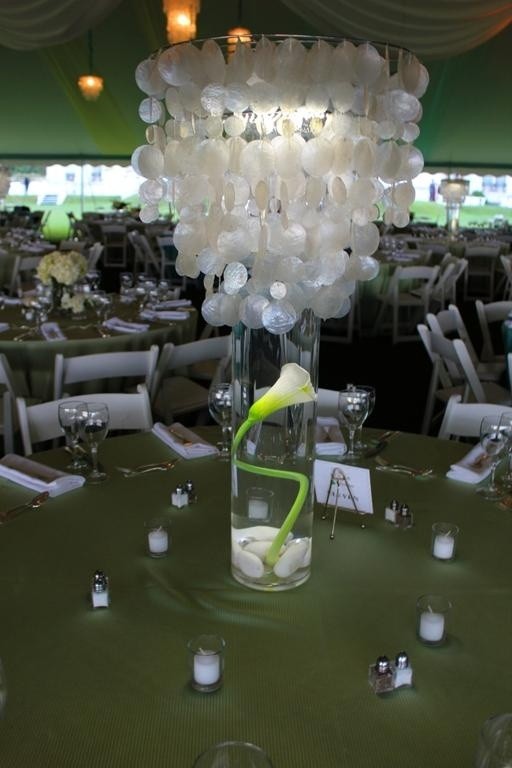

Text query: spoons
(354, 430), (401, 451)
(0, 491), (51, 524)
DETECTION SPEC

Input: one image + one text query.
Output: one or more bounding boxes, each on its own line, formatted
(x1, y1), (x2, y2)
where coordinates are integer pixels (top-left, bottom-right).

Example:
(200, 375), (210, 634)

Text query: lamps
(78, 30), (104, 103)
(226, 1), (252, 61)
(162, 1), (201, 47)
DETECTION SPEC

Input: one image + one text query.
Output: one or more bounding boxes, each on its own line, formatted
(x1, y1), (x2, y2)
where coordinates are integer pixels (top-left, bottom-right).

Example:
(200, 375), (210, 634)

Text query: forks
(322, 425), (333, 443)
(469, 459), (483, 470)
(112, 458), (179, 481)
(374, 464), (434, 479)
(63, 322), (93, 330)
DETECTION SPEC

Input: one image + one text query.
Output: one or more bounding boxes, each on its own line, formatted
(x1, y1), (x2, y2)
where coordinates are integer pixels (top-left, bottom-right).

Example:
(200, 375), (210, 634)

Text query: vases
(229, 307), (321, 592)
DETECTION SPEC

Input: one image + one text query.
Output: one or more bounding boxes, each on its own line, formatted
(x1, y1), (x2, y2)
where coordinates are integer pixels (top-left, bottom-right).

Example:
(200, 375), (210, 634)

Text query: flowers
(230, 361), (318, 568)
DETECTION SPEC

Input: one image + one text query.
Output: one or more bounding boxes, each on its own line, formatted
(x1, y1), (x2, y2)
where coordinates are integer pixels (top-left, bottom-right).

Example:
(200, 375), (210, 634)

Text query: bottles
(385, 500), (415, 532)
(391, 649), (413, 692)
(171, 480), (198, 508)
(368, 652), (395, 696)
(89, 569), (111, 610)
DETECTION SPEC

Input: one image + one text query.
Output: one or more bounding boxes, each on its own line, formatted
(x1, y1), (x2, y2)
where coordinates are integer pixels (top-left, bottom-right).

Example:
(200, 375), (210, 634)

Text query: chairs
(0, 203), (512, 471)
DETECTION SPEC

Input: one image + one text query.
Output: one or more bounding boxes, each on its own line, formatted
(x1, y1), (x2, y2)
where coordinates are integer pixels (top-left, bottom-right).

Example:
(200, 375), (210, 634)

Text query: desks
(0, 418), (512, 768)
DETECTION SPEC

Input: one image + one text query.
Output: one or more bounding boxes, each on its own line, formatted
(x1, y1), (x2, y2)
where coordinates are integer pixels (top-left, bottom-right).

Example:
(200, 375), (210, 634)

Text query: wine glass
(209, 385), (232, 462)
(474, 411), (511, 512)
(18, 270), (172, 327)
(337, 384), (376, 460)
(57, 401), (89, 471)
(75, 402), (110, 484)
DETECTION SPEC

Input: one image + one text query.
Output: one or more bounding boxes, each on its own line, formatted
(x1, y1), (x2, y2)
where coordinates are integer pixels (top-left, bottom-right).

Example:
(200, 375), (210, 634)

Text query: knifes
(114, 322), (141, 330)
(65, 442), (104, 473)
(167, 425), (192, 445)
(13, 330), (34, 342)
(95, 325), (112, 338)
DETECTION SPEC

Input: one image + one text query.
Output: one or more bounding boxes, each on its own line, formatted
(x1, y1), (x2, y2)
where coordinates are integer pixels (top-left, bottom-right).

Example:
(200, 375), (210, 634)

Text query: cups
(244, 488), (275, 523)
(142, 515), (175, 557)
(380, 235), (407, 257)
(429, 522), (459, 560)
(183, 634), (227, 693)
(191, 742), (273, 768)
(10, 228), (33, 248)
(473, 711), (511, 767)
(414, 591), (452, 648)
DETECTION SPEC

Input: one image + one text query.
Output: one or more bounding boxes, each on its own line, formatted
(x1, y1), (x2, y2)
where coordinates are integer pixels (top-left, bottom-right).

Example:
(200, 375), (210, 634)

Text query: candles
(431, 527), (458, 559)
(147, 524), (167, 552)
(421, 605), (443, 639)
(192, 645), (218, 683)
